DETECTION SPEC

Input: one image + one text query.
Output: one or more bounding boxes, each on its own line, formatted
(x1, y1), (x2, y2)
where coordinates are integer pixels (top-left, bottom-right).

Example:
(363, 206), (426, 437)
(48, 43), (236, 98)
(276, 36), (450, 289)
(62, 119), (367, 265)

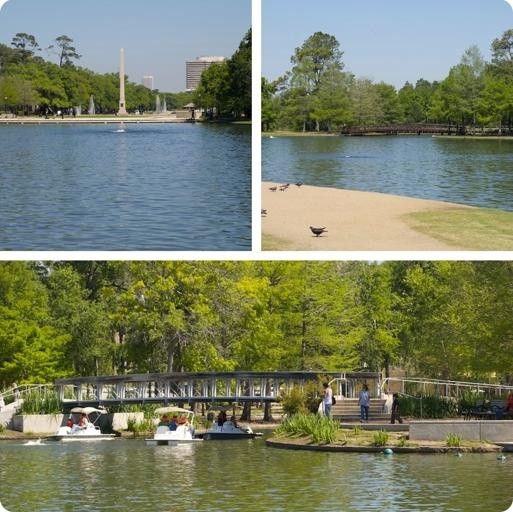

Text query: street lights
(3, 94), (8, 119)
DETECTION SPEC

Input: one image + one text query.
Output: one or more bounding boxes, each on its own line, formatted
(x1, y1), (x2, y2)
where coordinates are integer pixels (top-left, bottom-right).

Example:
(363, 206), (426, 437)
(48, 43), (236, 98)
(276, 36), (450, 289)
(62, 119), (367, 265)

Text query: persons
(357, 384), (371, 422)
(323, 382), (333, 420)
(390, 393), (403, 424)
(158, 414), (186, 425)
(67, 413), (89, 427)
(218, 413), (228, 426)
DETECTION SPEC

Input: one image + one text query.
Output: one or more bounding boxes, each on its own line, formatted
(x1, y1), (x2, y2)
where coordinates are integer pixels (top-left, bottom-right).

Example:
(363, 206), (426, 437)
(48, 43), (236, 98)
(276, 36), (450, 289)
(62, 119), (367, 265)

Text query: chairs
(460, 401), (513, 420)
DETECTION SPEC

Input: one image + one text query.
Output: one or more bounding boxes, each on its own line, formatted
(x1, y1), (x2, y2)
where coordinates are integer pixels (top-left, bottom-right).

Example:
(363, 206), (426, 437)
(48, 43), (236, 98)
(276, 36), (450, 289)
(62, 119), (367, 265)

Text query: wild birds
(268, 183), (291, 193)
(260, 208), (267, 215)
(308, 226), (327, 237)
(295, 181), (304, 188)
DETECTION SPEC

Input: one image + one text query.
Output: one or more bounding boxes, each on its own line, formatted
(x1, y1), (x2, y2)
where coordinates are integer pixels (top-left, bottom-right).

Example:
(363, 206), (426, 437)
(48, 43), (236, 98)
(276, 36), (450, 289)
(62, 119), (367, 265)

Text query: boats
(144, 405), (203, 447)
(194, 404), (264, 441)
(45, 407), (117, 443)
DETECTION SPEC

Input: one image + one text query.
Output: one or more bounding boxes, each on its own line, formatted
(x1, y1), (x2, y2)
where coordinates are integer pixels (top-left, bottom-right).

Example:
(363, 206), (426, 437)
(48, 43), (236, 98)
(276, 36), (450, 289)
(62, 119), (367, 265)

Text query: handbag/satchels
(332, 396), (336, 405)
